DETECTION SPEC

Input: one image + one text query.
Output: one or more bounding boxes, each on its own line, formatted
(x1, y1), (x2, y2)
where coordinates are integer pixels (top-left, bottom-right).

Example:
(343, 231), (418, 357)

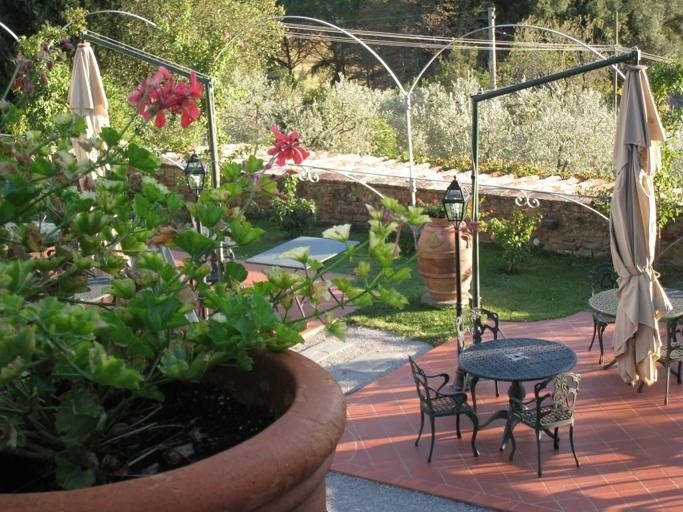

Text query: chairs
(408, 356), (478, 463)
(588, 262), (619, 364)
(637, 312), (683, 406)
(506, 371), (582, 478)
(455, 307), (499, 413)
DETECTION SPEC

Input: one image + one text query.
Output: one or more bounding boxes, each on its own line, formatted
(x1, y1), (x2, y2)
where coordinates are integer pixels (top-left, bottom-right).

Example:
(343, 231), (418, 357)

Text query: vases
(0, 331), (347, 512)
(430, 217), (448, 227)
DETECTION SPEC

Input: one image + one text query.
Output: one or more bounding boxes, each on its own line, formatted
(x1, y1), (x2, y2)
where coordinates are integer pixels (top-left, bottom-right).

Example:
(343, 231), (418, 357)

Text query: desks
(245, 234), (361, 328)
(458, 337), (578, 452)
(587, 287), (683, 370)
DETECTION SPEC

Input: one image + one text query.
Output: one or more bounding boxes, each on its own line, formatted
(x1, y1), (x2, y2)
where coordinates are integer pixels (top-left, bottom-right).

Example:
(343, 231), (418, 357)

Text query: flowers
(416, 197), (495, 218)
(0, 6), (479, 490)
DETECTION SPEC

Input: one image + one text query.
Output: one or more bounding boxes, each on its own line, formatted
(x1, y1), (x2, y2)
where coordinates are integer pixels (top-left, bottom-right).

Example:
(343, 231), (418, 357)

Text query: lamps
(183, 150), (207, 234)
(442, 176), (466, 356)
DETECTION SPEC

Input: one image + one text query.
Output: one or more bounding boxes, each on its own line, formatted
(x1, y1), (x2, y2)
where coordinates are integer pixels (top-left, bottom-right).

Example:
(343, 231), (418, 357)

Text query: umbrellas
(468, 46), (673, 388)
(66, 28), (224, 268)
